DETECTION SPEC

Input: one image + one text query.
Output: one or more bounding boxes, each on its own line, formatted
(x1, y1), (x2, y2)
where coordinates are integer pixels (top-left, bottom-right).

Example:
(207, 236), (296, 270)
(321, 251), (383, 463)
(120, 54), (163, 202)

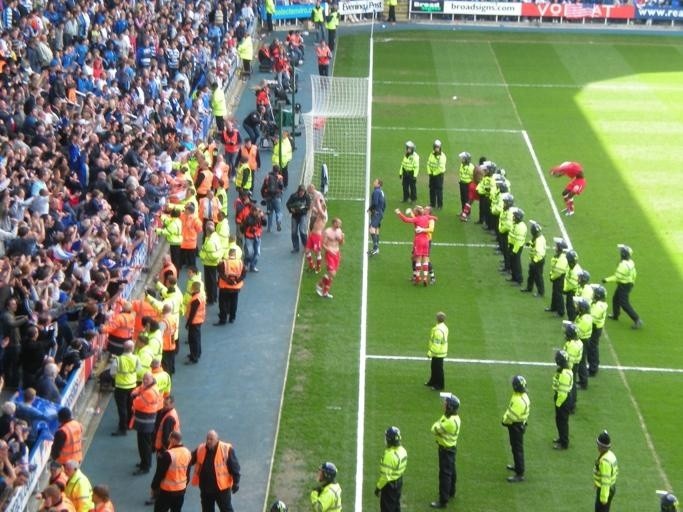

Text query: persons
(265, 0), (275, 29)
(213, 155), (228, 187)
(147, 361), (170, 403)
(155, 396), (179, 448)
(214, 181), (228, 216)
(236, 156), (253, 198)
(154, 254), (176, 283)
(107, 302), (135, 349)
(497, 195), (513, 271)
(326, 5), (337, 47)
(200, 190), (221, 228)
(185, 266), (202, 292)
(564, 320), (583, 379)
(236, 206), (266, 273)
(311, 1), (325, 42)
(162, 287), (180, 327)
(317, 40), (332, 88)
(90, 486), (111, 507)
(585, 283), (606, 375)
(150, 432), (191, 506)
(230, 0), (260, 39)
(471, 155), (512, 250)
(397, 205), (428, 286)
(602, 243), (644, 329)
(222, 121), (242, 182)
(306, 186), (328, 229)
(156, 306), (177, 373)
(405, 204), (436, 283)
(195, 431), (239, 507)
(0, 387), (51, 501)
(40, 486), (70, 506)
(59, 460), (91, 506)
(49, 409), (82, 470)
(151, 209), (181, 274)
(133, 373), (162, 477)
(303, 197), (326, 274)
(426, 313), (448, 388)
(236, 139), (260, 180)
(309, 463), (342, 512)
(519, 221), (547, 295)
(236, 191), (255, 230)
(181, 282), (206, 364)
(199, 225), (220, 305)
(213, 212), (229, 256)
(243, 107), (263, 142)
(216, 248), (246, 327)
(562, 246), (585, 318)
(153, 273), (179, 302)
(507, 208), (526, 286)
(132, 334), (152, 382)
(314, 219), (345, 299)
(169, 188), (198, 220)
(428, 139), (446, 208)
(141, 318), (162, 367)
(262, 166), (285, 232)
(572, 271), (592, 315)
(109, 340), (142, 435)
(575, 299), (594, 382)
(137, 288), (159, 326)
(208, 83), (226, 131)
(287, 185), (309, 253)
(502, 375), (530, 481)
(458, 153), (474, 222)
(0, 85), (148, 290)
(369, 180), (385, 256)
(399, 140), (419, 201)
(554, 351), (577, 448)
(0, 287), (108, 400)
(228, 236), (243, 262)
(269, 502), (284, 511)
(145, 86), (211, 141)
(2, 0), (234, 91)
(177, 203), (204, 272)
(234, 29), (305, 104)
(272, 131), (293, 183)
(592, 431), (617, 512)
(656, 491), (678, 511)
(169, 136), (212, 187)
(544, 237), (570, 313)
(373, 427), (407, 511)
(141, 140), (185, 204)
(431, 392), (461, 508)
(549, 162), (586, 215)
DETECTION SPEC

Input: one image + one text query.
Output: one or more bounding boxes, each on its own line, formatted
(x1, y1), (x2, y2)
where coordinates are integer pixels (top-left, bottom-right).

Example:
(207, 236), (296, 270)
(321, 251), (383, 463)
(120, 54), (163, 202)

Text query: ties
(209, 200), (212, 220)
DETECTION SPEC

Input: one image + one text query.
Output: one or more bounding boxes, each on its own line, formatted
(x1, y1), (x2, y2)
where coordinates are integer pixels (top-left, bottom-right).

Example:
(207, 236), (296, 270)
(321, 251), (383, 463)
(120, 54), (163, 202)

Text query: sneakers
(505, 277), (517, 282)
(206, 296), (218, 307)
(246, 265), (249, 271)
(368, 247), (379, 257)
(456, 211), (464, 216)
(474, 219), (483, 224)
(553, 438), (562, 443)
(278, 224), (282, 232)
(520, 288), (532, 293)
(250, 266), (259, 272)
(576, 384), (587, 390)
(424, 277), (429, 287)
(553, 444), (568, 449)
(430, 278), (435, 286)
(588, 371), (595, 376)
(459, 215), (468, 222)
(566, 210), (575, 216)
(409, 199), (417, 205)
(315, 267), (322, 274)
(291, 248), (300, 253)
(411, 276), (417, 281)
(317, 286), (324, 297)
(506, 473), (524, 481)
(425, 381), (433, 387)
(494, 237), (508, 273)
(324, 293), (334, 298)
(436, 207), (443, 210)
(568, 408), (576, 415)
(609, 314), (619, 320)
(533, 292), (544, 297)
(429, 204), (435, 208)
(399, 198), (409, 202)
(306, 266), (316, 271)
(554, 313), (564, 318)
(431, 501), (448, 508)
(561, 207), (568, 213)
(413, 276), (422, 285)
(506, 465), (517, 470)
(266, 226), (273, 232)
(483, 224), (497, 235)
(432, 385), (444, 391)
(545, 306), (557, 312)
(631, 320), (643, 329)
(512, 284), (522, 287)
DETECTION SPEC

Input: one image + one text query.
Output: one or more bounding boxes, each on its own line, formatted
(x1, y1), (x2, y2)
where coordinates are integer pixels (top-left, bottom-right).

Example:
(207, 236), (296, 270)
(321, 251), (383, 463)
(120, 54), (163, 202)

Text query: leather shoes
(137, 463), (146, 468)
(186, 354), (192, 358)
(185, 359), (198, 365)
(112, 427), (128, 436)
(135, 468), (149, 477)
(146, 495), (159, 505)
(185, 340), (191, 344)
(229, 318), (236, 323)
(212, 319), (228, 326)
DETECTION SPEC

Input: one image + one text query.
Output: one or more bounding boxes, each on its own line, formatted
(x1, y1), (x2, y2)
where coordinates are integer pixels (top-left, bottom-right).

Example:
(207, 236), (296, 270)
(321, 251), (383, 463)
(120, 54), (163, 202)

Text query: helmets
(574, 297), (589, 313)
(591, 285), (607, 301)
(501, 192), (513, 206)
(271, 500), (287, 512)
(618, 244), (633, 259)
(554, 349), (570, 369)
(440, 392), (460, 415)
(554, 237), (567, 253)
(459, 152), (471, 164)
(433, 140), (441, 150)
(511, 206), (523, 222)
(563, 320), (580, 338)
(383, 426), (401, 447)
(564, 248), (578, 264)
(407, 141), (416, 152)
(531, 221), (542, 238)
(578, 269), (589, 284)
(512, 376), (527, 392)
(483, 161), (496, 176)
(318, 462), (337, 481)
(495, 181), (508, 193)
(494, 175), (505, 181)
(657, 490), (679, 512)
(550, 166), (564, 176)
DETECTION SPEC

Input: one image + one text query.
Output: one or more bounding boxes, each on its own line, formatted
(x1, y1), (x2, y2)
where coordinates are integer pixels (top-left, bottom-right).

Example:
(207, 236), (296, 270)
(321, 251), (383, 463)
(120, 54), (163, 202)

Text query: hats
(253, 208), (261, 213)
(274, 165), (280, 171)
(298, 185), (305, 191)
(252, 199), (258, 203)
(596, 430), (610, 448)
